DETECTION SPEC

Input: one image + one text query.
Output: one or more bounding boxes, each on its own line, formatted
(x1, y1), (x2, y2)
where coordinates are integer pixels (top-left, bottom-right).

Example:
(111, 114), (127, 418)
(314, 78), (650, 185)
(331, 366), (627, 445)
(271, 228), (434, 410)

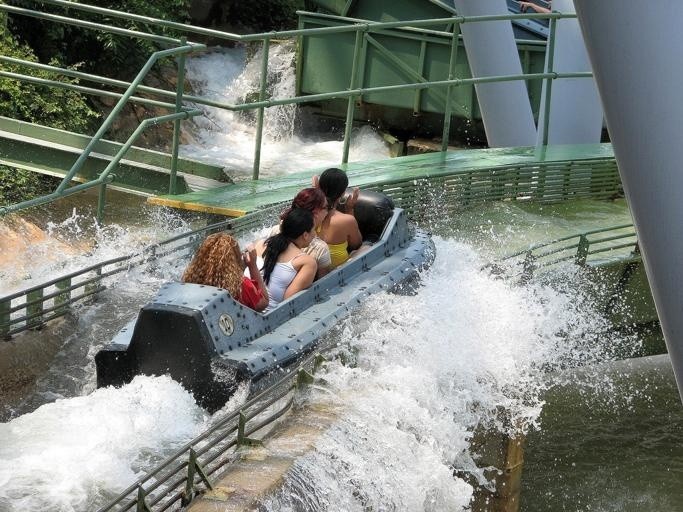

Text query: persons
(520, 1), (552, 13)
(312, 168), (373, 278)
(241, 207), (317, 314)
(182, 231), (270, 310)
(254, 187), (331, 279)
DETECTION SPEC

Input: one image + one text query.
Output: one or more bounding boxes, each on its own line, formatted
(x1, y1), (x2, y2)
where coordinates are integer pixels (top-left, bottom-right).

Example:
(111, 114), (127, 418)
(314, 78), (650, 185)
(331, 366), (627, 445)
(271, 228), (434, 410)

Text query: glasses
(322, 205), (328, 209)
